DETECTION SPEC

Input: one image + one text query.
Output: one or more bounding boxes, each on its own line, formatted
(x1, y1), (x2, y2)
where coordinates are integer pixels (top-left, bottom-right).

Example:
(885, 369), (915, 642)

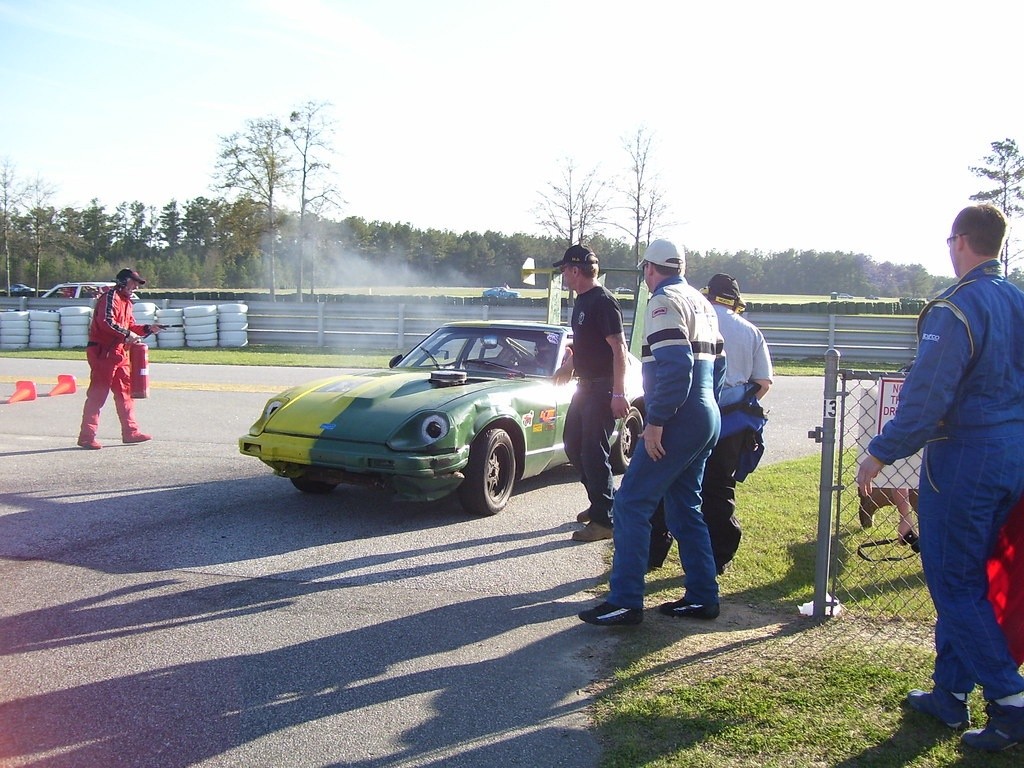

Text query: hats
(552, 243), (600, 267)
(116, 267), (146, 285)
(708, 273), (739, 308)
(636, 238), (687, 268)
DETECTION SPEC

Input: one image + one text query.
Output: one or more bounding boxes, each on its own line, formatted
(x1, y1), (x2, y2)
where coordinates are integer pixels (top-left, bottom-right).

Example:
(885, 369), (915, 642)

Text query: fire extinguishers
(130, 323), (184, 399)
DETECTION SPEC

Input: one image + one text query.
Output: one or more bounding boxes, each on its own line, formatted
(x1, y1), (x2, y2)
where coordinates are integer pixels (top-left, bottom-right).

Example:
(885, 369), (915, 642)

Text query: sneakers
(960, 700), (1024, 764)
(908, 674), (973, 729)
(578, 601), (644, 626)
(659, 598), (720, 620)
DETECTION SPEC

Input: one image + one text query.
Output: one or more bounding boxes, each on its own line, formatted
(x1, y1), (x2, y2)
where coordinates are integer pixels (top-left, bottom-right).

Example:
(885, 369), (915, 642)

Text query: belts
(577, 376), (614, 385)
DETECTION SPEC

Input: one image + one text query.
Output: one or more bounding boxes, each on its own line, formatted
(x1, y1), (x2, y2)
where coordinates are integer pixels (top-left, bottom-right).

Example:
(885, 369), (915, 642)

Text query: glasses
(559, 263), (580, 272)
(640, 262), (657, 277)
(946, 230), (972, 248)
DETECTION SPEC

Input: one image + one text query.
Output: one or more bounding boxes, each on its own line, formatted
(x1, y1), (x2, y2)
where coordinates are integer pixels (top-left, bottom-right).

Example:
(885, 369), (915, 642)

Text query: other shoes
(577, 509), (590, 523)
(572, 520), (614, 541)
(650, 529), (674, 569)
(77, 439), (102, 449)
(123, 431), (152, 443)
(714, 556), (725, 574)
(857, 487), (876, 528)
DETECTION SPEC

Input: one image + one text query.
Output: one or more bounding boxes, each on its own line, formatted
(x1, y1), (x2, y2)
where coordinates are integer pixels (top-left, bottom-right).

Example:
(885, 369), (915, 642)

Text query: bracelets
(612, 394), (624, 398)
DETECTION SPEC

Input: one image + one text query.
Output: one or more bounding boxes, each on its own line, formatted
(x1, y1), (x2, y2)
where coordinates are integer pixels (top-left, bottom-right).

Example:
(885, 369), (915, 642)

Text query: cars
(239, 320), (647, 516)
(41, 282), (139, 300)
(483, 287), (519, 300)
(838, 293), (853, 299)
(6, 283), (36, 292)
(615, 287), (631, 291)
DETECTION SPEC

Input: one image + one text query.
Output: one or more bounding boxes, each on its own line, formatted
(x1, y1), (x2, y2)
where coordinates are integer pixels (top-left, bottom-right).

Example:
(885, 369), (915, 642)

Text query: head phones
(110, 278), (128, 287)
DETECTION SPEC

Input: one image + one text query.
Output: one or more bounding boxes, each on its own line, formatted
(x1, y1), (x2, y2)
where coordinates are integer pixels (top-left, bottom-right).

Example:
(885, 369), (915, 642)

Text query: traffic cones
(6, 381), (36, 404)
(47, 375), (77, 396)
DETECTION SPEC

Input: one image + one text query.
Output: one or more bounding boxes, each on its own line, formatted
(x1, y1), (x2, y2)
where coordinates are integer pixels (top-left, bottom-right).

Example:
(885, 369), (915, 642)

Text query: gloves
(127, 331), (142, 343)
(148, 324), (165, 334)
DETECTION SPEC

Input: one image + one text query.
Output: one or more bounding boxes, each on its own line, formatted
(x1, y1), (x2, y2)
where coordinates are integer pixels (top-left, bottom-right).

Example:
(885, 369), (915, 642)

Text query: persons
(579, 239), (727, 626)
(856, 205), (1024, 751)
(78, 268), (161, 449)
(551, 245), (631, 542)
(534, 340), (572, 377)
(649, 273), (773, 575)
